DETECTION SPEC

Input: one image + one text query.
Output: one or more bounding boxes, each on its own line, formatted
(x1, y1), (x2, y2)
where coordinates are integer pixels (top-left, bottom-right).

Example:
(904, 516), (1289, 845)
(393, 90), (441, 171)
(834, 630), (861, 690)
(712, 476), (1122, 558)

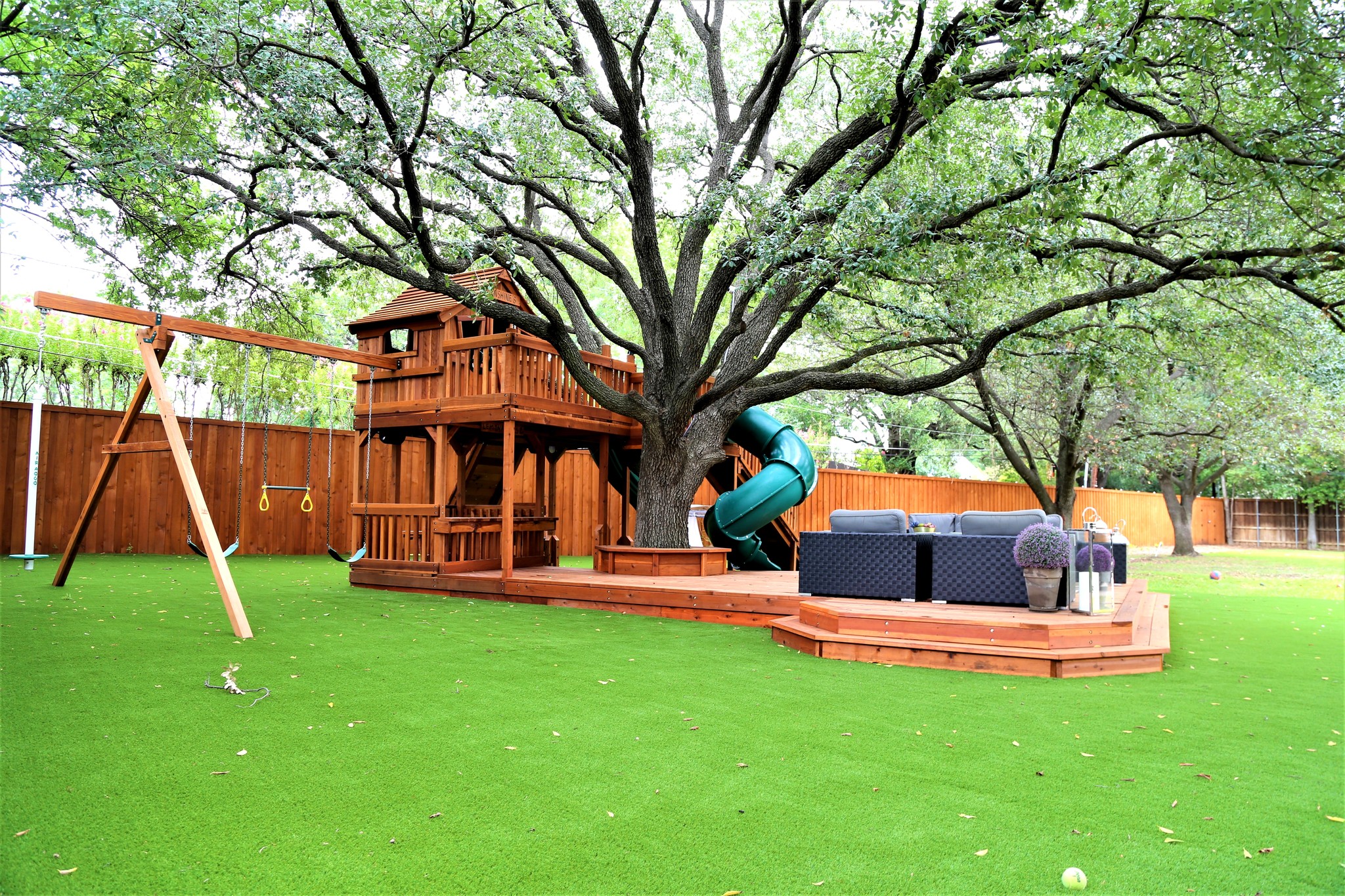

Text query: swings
(326, 357), (376, 563)
(259, 346), (321, 513)
(186, 332), (255, 559)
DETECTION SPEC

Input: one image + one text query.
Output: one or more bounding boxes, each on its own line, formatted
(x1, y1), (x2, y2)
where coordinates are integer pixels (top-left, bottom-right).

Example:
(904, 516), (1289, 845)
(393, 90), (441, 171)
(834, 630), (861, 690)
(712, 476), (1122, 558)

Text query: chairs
(797, 504), (1085, 612)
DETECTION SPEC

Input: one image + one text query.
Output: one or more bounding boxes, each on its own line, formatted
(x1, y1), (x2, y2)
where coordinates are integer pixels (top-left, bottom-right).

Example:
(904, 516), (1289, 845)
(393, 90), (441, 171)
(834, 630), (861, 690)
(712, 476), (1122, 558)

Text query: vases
(1100, 570), (1112, 583)
(1021, 565), (1064, 611)
(913, 526), (936, 532)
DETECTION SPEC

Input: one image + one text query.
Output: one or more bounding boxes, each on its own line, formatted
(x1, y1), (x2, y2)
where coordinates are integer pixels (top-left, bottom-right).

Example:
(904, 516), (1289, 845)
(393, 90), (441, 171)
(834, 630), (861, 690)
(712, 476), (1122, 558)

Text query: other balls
(1210, 570), (1222, 581)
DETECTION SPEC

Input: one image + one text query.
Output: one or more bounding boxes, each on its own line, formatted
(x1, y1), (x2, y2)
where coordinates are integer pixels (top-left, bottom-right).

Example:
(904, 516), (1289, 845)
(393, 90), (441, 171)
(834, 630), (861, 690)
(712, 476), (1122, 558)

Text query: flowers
(1103, 556), (1115, 572)
(1010, 521), (1074, 571)
(909, 521), (937, 529)
(1074, 542), (1115, 572)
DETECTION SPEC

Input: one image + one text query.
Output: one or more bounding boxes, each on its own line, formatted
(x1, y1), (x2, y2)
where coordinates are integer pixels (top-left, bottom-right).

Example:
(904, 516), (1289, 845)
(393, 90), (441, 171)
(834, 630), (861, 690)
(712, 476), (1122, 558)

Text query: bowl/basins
(913, 526), (936, 533)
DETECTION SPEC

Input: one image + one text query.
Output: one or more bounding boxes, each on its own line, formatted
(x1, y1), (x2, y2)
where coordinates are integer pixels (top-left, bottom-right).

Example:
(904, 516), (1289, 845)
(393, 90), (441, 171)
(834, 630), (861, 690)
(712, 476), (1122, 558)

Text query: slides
(703, 404), (819, 573)
(588, 447), (640, 511)
